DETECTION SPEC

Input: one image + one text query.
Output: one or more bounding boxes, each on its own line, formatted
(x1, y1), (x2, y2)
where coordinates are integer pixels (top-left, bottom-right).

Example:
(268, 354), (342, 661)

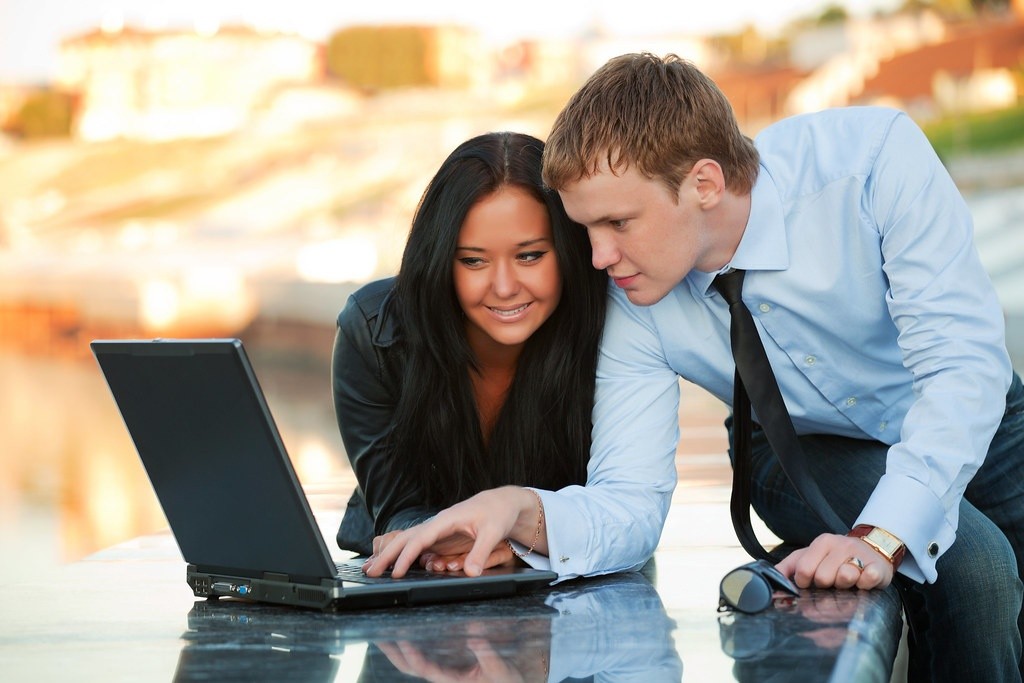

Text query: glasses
(717, 558), (801, 615)
(718, 600), (798, 663)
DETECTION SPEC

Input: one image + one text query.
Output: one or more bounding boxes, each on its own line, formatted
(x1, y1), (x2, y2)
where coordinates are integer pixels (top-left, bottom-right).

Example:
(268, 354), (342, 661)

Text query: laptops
(90, 338), (560, 618)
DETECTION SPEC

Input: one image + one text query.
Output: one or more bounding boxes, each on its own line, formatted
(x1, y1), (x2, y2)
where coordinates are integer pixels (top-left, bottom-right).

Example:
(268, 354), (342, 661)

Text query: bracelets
(504, 488), (542, 557)
(538, 648), (549, 683)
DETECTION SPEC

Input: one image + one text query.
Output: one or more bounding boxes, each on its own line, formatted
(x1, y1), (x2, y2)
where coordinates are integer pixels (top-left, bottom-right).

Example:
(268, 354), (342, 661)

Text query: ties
(713, 269), (921, 649)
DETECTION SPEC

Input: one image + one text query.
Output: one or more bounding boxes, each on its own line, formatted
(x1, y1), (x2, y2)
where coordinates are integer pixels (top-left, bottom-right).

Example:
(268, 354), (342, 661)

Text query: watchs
(845, 526), (904, 572)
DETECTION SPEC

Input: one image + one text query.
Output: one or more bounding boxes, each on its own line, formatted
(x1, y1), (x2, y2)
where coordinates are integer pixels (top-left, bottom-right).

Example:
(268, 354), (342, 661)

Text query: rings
(846, 557), (864, 570)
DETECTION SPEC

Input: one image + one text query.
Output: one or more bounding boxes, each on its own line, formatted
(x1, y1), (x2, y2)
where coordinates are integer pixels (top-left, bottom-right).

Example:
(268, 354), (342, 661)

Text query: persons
(330, 132), (608, 573)
(362, 55), (1024, 683)
(356, 571), (859, 683)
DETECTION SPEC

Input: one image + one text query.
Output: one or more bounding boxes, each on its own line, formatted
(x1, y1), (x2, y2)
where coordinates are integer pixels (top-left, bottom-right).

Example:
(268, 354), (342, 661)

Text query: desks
(0, 483), (903, 683)
